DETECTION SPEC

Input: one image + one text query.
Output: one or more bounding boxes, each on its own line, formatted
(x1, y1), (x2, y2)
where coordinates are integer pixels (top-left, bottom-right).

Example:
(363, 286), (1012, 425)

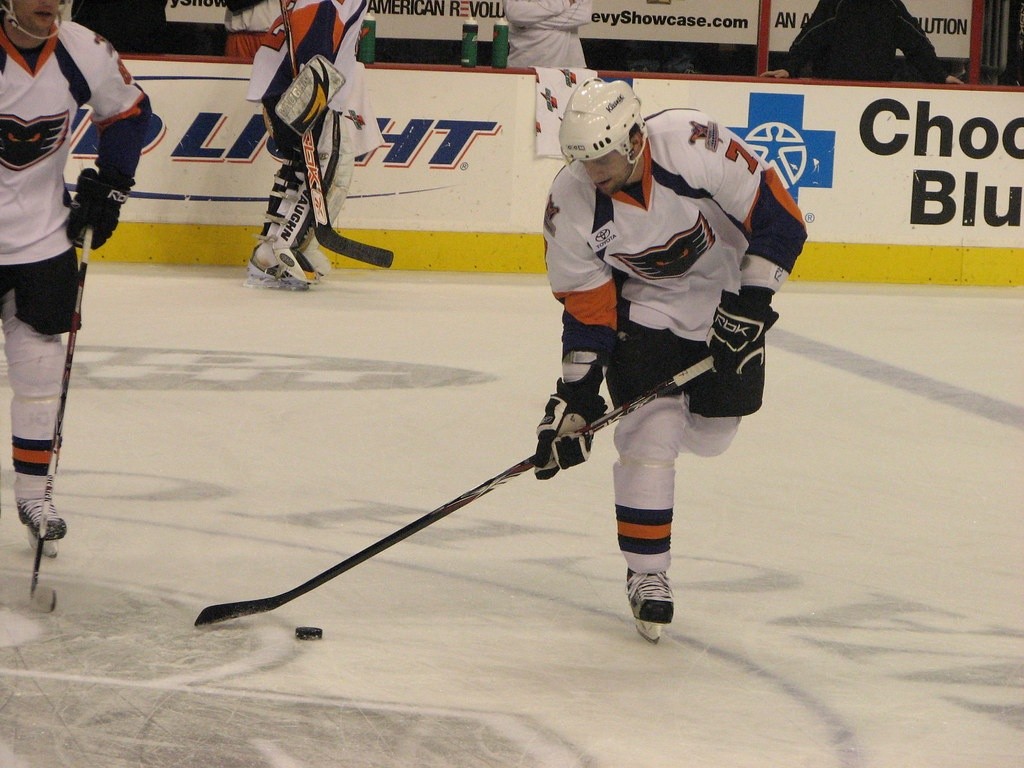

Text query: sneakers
(243, 253), (309, 291)
(16, 497), (67, 558)
(626, 568), (674, 643)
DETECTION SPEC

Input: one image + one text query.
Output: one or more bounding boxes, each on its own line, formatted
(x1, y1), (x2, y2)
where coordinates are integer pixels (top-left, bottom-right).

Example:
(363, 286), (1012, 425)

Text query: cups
(461, 17), (478, 66)
(357, 13), (377, 64)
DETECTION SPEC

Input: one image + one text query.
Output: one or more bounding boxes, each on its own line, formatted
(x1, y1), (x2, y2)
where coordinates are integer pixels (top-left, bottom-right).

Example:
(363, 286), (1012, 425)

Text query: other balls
(295, 627), (324, 642)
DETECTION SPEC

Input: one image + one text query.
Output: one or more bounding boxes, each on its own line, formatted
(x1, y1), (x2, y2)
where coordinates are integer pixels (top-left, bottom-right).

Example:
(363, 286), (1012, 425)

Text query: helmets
(66, 169), (136, 250)
(559, 76), (647, 161)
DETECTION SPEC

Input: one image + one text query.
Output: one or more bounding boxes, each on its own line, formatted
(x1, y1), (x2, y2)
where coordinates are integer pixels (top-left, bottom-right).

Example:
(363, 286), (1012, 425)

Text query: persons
(224, 0), (283, 58)
(0, 0), (149, 558)
(502, 0), (593, 68)
(535, 78), (808, 642)
(760, 0), (964, 84)
(245, 0), (371, 290)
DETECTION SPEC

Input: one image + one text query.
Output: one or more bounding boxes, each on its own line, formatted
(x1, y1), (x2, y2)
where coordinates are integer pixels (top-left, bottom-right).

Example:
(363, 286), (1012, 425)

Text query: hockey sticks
(192, 357), (715, 627)
(279, 0), (395, 270)
(28, 229), (95, 614)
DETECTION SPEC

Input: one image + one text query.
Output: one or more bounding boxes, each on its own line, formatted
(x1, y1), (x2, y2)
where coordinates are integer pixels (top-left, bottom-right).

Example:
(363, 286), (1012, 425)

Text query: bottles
(491, 17), (508, 67)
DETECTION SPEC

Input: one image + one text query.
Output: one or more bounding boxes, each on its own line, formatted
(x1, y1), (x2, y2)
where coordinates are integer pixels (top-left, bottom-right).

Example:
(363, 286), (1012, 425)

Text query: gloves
(533, 377), (607, 480)
(706, 288), (779, 374)
